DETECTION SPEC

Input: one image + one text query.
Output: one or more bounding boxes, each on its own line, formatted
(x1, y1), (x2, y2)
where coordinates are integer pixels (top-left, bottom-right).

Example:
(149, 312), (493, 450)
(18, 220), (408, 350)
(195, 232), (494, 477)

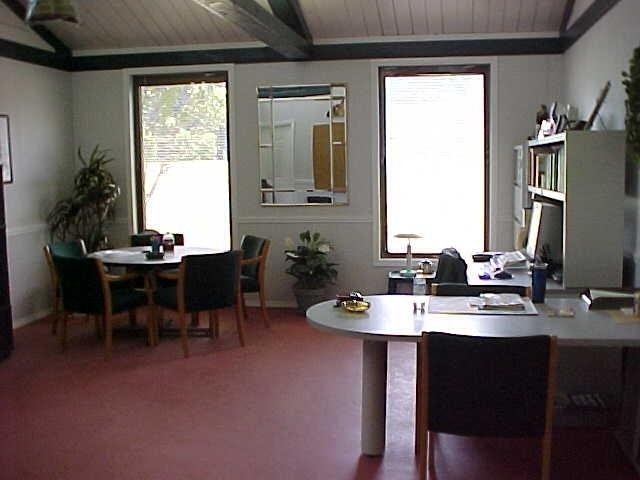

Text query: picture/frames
(0, 114), (13, 184)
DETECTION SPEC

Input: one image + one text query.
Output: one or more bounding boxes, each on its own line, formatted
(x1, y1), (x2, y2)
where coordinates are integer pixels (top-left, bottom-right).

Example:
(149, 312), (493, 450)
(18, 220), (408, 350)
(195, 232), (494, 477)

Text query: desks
(462, 245), (566, 295)
(306, 294), (640, 458)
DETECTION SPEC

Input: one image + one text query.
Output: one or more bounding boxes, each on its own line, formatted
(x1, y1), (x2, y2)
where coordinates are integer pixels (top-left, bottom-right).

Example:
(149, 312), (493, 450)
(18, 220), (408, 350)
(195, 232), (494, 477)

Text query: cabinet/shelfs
(523, 130), (625, 292)
(387, 271), (436, 296)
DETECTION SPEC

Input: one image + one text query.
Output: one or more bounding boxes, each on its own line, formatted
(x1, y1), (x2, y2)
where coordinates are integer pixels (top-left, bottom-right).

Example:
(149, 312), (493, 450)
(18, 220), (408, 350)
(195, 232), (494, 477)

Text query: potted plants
(283, 229), (340, 314)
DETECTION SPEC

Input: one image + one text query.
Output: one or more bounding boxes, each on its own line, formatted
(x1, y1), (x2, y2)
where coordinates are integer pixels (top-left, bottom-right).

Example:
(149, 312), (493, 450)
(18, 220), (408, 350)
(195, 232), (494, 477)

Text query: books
(528, 148), (564, 192)
(476, 294), (527, 310)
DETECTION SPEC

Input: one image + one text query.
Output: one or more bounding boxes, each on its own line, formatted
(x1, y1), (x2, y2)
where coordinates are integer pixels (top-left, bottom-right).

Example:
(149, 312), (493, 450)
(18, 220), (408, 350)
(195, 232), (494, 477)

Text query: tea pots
(420, 258), (433, 274)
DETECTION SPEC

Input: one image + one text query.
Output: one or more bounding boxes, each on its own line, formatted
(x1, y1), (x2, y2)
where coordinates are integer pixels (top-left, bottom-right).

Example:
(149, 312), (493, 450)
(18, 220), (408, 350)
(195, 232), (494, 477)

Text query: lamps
(394, 234), (424, 266)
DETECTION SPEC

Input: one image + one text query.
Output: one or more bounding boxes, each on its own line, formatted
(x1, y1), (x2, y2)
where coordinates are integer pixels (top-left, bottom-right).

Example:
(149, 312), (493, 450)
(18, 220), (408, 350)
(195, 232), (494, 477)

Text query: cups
(529, 263), (549, 304)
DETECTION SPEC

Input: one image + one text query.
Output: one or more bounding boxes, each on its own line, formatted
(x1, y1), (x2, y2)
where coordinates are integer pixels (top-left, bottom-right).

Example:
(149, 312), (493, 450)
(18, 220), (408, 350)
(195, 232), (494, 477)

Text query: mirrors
(257, 83), (348, 207)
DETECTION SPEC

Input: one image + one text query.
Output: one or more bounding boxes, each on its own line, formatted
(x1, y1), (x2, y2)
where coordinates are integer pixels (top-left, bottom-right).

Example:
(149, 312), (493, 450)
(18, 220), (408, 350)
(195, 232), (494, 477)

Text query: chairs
(415, 329), (559, 480)
(43, 239), (148, 337)
(151, 249), (247, 358)
(432, 247), (483, 295)
(52, 254), (151, 364)
(237, 233), (272, 329)
